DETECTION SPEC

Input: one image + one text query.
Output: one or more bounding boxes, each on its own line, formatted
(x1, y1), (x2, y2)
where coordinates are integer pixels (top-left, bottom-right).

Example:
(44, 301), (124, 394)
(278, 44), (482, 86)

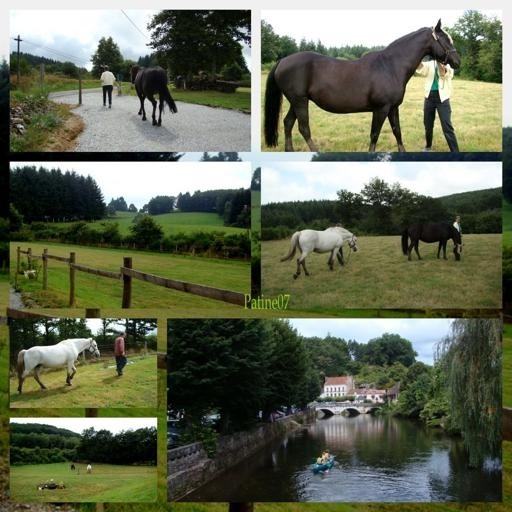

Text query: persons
(113, 332), (126, 375)
(415, 59), (460, 152)
(316, 449), (330, 465)
(451, 215), (463, 236)
(100, 65), (116, 109)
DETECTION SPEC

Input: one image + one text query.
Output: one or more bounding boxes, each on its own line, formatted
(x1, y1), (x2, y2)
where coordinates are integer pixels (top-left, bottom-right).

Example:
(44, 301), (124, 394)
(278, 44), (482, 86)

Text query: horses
(129, 65), (178, 127)
(280, 227), (358, 280)
(17, 338), (100, 395)
(264, 18), (461, 152)
(401, 217), (466, 261)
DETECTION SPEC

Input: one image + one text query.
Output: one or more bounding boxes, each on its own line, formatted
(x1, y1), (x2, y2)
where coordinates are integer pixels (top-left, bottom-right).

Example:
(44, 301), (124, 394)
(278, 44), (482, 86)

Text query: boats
(313, 454), (336, 474)
